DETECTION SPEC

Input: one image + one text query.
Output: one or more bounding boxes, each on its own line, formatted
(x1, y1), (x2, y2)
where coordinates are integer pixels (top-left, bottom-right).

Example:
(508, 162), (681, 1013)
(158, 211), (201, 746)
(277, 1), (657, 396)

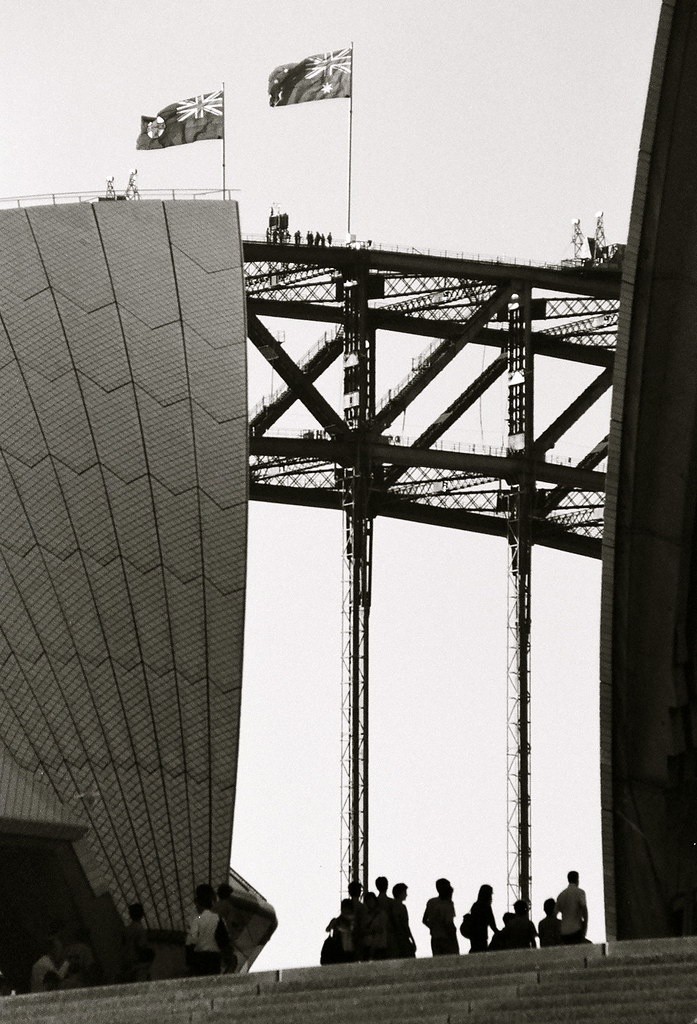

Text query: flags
(136, 90), (225, 151)
(267, 47), (351, 107)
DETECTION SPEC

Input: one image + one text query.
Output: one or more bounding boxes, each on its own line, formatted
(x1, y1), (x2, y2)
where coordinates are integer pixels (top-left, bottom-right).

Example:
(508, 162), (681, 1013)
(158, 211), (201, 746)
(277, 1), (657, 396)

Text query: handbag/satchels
(460, 913), (473, 938)
(214, 916), (231, 946)
(320, 933), (340, 964)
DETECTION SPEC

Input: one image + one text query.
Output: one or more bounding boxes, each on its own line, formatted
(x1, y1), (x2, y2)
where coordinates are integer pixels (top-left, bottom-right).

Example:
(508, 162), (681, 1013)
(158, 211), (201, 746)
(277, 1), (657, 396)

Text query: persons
(470, 870), (590, 950)
(324, 876), (417, 960)
(266, 227), (332, 246)
(184, 883), (237, 976)
(28, 940), (80, 994)
(422, 879), (461, 957)
(118, 899), (155, 983)
